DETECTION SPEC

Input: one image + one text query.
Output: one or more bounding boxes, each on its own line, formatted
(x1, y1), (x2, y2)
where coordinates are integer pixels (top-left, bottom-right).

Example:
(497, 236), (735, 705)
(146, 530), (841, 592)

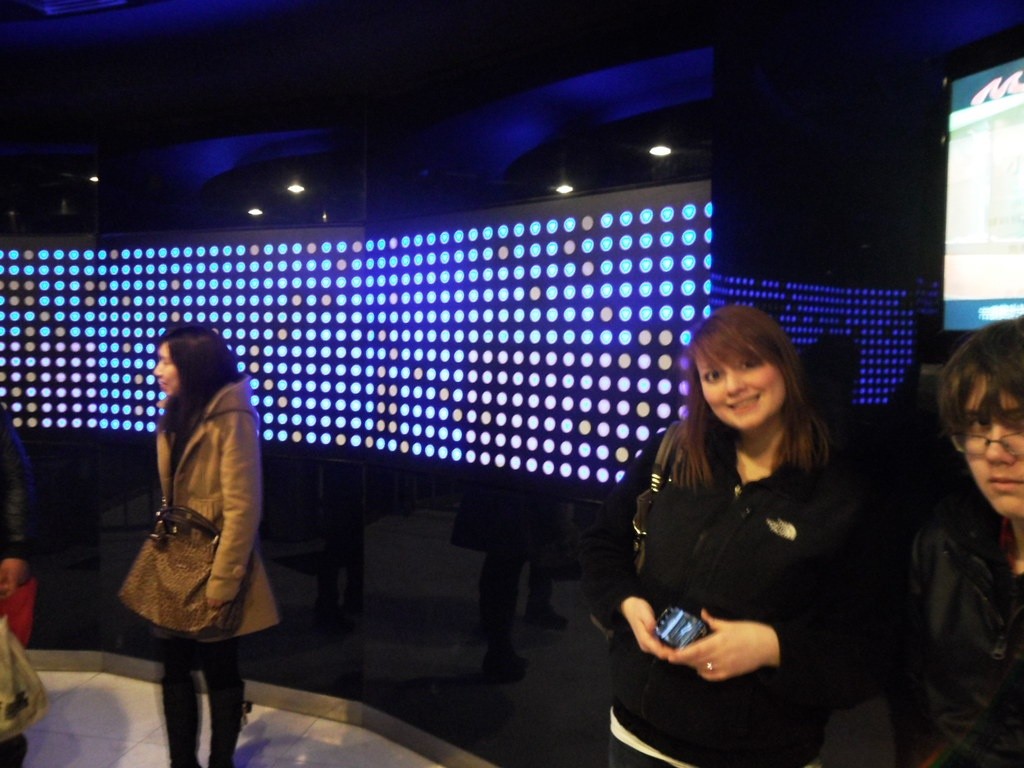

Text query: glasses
(949, 429), (1024, 457)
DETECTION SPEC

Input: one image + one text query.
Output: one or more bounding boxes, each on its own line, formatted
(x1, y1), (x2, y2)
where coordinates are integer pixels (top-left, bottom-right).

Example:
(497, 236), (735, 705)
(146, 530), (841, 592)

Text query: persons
(153, 323), (281, 768)
(888, 319), (1024, 768)
(579, 306), (886, 768)
(0, 407), (44, 768)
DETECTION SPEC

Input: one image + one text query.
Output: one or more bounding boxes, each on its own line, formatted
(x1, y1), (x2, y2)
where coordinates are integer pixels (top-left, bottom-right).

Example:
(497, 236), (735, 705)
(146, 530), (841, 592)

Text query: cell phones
(653, 605), (711, 649)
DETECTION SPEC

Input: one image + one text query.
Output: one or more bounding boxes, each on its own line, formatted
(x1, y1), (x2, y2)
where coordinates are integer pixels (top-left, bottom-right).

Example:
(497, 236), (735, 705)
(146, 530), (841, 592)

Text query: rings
(707, 662), (713, 671)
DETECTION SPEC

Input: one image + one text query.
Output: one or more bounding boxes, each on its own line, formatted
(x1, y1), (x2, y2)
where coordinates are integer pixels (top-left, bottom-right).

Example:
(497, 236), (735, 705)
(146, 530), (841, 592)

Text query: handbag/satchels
(115, 505), (256, 642)
(0, 616), (51, 742)
(590, 421), (688, 643)
